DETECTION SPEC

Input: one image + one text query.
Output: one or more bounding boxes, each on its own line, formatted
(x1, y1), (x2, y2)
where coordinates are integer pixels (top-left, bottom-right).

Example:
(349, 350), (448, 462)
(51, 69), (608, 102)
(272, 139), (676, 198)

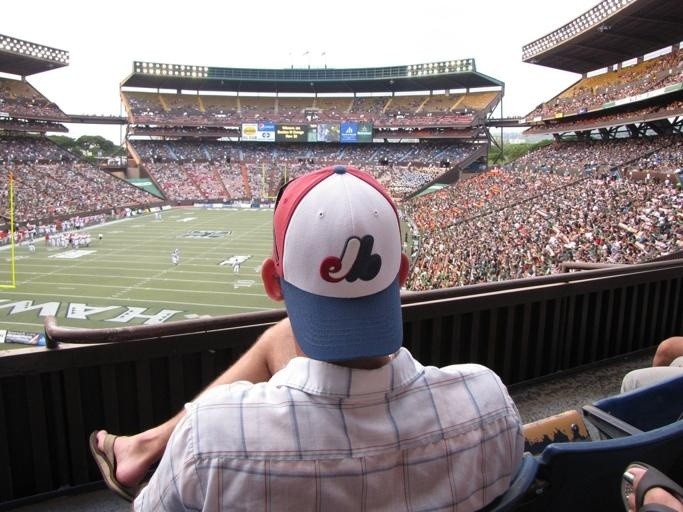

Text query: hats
(272, 164), (404, 363)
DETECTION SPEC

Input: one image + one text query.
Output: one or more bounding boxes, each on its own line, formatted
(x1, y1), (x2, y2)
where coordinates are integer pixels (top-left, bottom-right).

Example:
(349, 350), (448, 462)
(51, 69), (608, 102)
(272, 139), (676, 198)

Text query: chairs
(486, 374), (683, 511)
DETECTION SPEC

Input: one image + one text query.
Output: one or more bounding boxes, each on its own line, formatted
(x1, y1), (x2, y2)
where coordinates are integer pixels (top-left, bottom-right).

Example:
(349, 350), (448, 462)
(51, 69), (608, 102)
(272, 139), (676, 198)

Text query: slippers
(90, 428), (138, 503)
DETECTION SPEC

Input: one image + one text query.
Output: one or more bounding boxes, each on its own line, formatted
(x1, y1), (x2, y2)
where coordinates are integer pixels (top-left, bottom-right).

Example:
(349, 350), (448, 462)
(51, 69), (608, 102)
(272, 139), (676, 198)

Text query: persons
(0, 49), (682, 295)
(618, 334), (682, 393)
(86, 162), (529, 511)
